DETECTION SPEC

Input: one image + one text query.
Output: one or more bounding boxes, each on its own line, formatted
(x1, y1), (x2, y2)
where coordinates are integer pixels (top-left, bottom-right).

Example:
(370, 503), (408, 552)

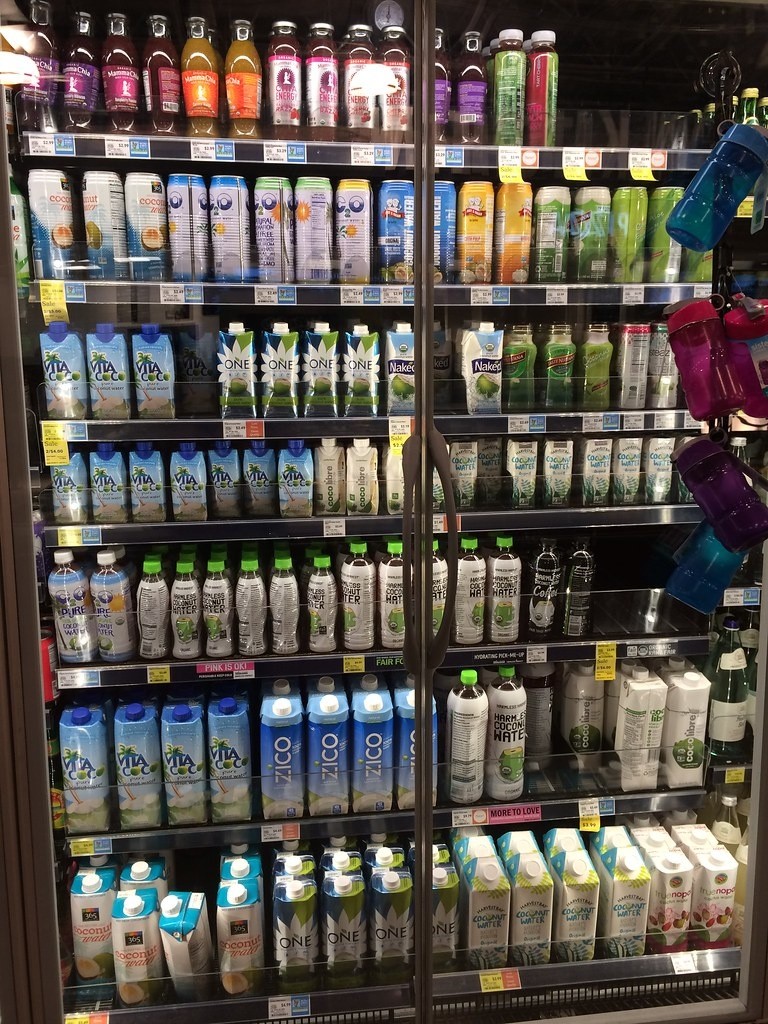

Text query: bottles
(666, 436), (768, 616)
(5, 88), (16, 135)
(181, 16), (219, 138)
(672, 254), (768, 421)
(703, 88), (768, 149)
(142, 15), (181, 136)
(101, 13), (142, 135)
(665, 123), (768, 253)
(225, 19), (263, 138)
(435, 28), (559, 147)
(702, 606), (761, 765)
(7, 162), (30, 282)
(48, 536), (592, 664)
(710, 792), (751, 947)
(264, 21), (411, 144)
(446, 663), (527, 803)
(502, 323), (614, 414)
(16, 0), (58, 131)
(656, 109), (706, 149)
(59, 10), (101, 133)
(207, 29), (230, 138)
